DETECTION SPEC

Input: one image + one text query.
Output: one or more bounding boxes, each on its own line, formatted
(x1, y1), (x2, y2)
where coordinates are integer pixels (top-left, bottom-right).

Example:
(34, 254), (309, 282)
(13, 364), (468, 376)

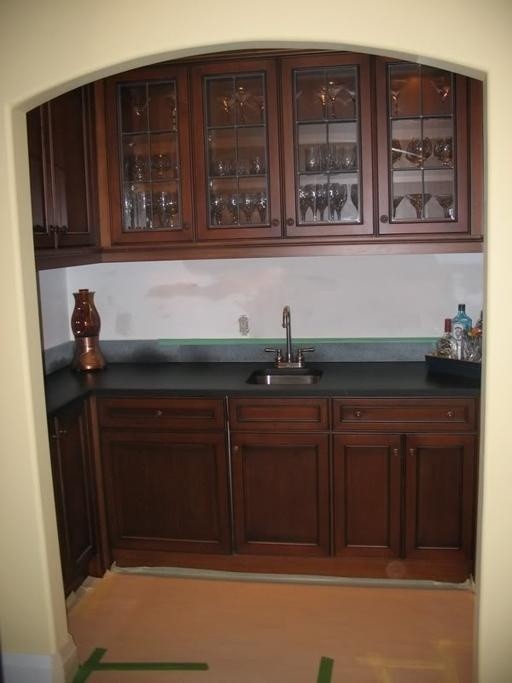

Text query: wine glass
(288, 78), (363, 227)
(125, 79), (180, 229)
(376, 77), (455, 228)
(204, 78), (269, 229)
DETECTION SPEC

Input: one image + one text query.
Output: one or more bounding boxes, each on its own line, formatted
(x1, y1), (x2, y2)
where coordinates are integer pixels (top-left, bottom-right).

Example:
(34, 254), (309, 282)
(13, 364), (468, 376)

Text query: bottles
(437, 303), (482, 359)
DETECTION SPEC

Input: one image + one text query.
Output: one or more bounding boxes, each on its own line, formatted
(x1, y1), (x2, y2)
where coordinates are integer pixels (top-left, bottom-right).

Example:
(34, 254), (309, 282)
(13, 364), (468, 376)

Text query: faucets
(282, 304), (295, 362)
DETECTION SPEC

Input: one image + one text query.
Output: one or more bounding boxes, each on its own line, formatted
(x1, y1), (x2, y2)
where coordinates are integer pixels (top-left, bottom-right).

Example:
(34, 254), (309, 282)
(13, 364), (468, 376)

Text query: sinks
(243, 369), (322, 386)
